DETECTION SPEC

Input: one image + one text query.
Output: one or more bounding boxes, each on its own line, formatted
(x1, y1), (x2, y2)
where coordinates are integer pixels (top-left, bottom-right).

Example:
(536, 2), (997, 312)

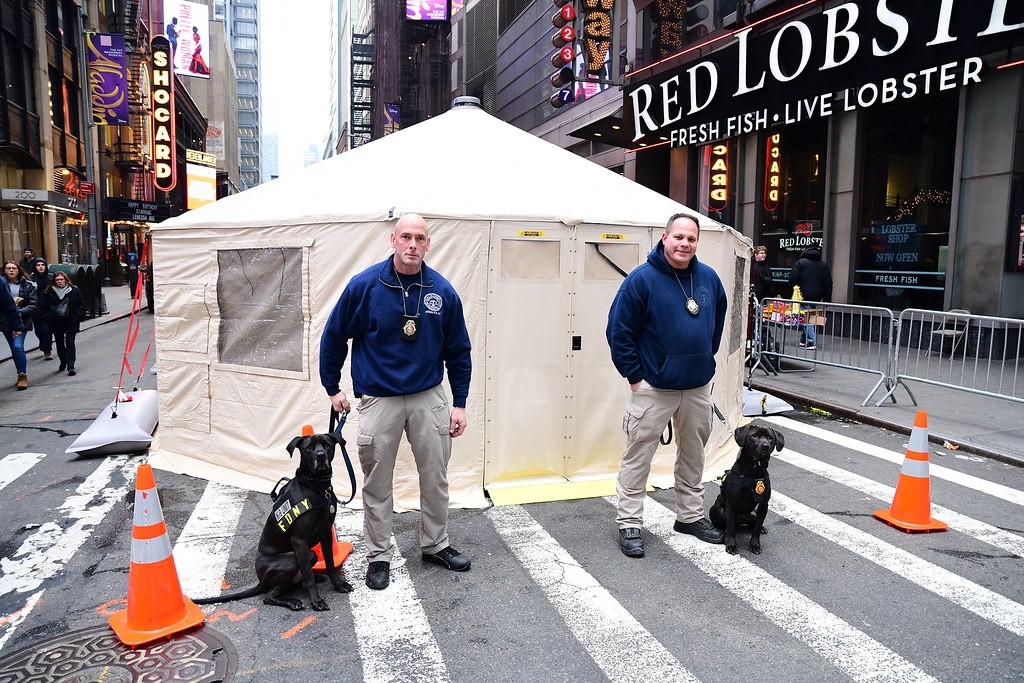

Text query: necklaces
(674, 272), (699, 315)
(393, 267), (423, 341)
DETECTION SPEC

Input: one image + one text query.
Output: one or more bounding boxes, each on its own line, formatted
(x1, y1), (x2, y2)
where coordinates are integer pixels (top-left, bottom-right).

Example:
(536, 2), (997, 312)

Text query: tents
(148, 96), (753, 514)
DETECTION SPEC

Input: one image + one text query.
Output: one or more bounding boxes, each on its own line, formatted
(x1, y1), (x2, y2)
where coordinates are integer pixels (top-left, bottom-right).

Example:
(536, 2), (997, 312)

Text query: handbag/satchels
(792, 285), (804, 314)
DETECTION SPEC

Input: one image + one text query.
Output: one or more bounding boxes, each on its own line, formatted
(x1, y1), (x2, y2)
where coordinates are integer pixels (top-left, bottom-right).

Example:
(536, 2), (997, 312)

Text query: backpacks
(760, 336), (781, 371)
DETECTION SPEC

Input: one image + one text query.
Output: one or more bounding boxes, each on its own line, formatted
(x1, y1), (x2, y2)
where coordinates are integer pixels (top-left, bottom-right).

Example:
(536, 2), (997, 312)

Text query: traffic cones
(300, 425), (354, 569)
(106, 464), (205, 650)
(873, 411), (948, 534)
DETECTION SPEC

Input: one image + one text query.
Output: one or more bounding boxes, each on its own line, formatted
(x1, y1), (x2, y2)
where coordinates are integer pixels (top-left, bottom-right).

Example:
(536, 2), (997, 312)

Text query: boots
(15, 374), (28, 388)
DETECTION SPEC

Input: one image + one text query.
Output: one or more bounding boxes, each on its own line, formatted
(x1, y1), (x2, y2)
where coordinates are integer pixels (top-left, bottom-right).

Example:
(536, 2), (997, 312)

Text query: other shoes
(44, 350), (53, 359)
(68, 368), (76, 374)
(59, 360), (67, 370)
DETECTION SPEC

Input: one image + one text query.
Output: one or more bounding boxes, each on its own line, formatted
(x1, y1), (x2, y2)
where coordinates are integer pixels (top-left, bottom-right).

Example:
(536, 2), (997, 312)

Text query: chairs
(924, 309), (970, 360)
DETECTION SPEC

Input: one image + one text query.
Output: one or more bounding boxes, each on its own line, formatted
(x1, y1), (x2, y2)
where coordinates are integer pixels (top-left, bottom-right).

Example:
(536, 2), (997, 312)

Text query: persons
(790, 244), (833, 350)
(753, 246), (773, 304)
(0, 260), (37, 392)
(605, 212), (727, 560)
(20, 248), (37, 273)
(167, 17), (182, 69)
(31, 256), (56, 361)
(189, 26), (209, 74)
(319, 212), (473, 590)
(47, 271), (84, 376)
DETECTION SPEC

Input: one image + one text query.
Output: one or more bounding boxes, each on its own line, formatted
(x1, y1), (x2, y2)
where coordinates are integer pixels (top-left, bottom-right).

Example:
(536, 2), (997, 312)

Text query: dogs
(190, 431), (354, 611)
(708, 425), (784, 556)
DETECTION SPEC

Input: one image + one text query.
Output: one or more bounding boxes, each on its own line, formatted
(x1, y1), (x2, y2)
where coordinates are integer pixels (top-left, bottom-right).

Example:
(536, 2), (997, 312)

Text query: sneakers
(674, 518), (726, 544)
(799, 339), (806, 346)
(366, 561), (390, 591)
(618, 528), (645, 556)
(800, 341), (816, 350)
(422, 547), (472, 571)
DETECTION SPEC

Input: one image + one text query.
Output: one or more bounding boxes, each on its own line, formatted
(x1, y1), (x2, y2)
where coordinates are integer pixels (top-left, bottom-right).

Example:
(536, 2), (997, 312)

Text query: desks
(754, 308), (817, 371)
